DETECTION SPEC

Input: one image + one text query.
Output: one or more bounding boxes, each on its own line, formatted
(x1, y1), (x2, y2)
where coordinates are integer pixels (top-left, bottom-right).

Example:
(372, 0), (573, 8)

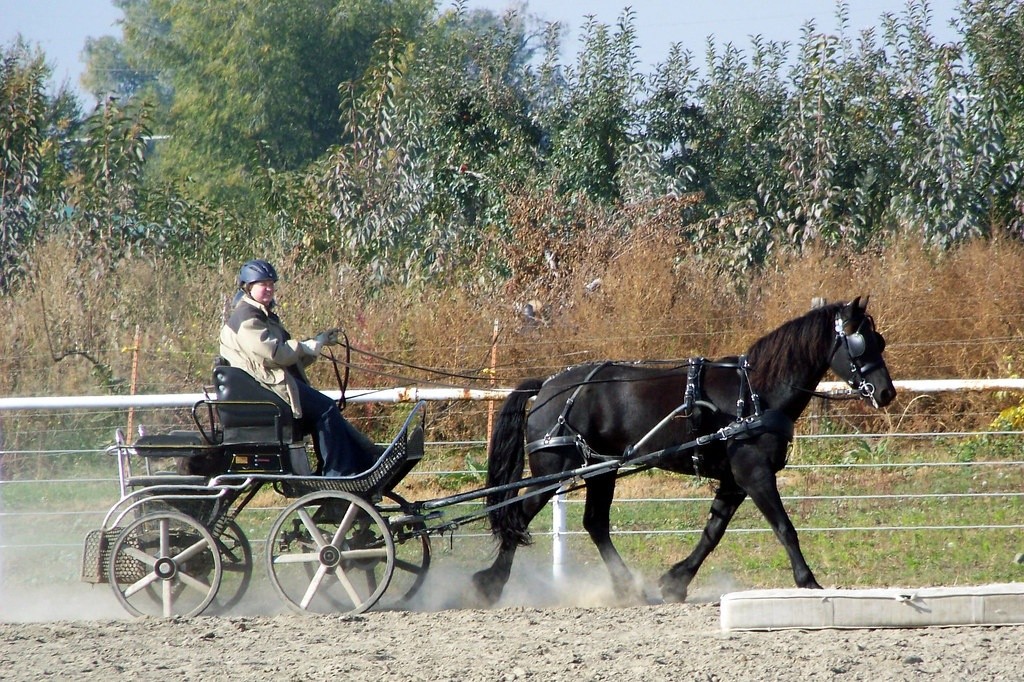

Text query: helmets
(237, 260), (279, 293)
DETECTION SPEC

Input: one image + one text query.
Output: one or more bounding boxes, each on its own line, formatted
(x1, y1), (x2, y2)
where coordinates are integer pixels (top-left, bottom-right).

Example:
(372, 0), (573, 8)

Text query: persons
(216, 258), (402, 480)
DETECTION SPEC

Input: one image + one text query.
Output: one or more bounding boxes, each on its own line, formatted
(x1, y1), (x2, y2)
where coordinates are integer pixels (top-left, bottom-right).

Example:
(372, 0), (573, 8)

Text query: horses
(472, 292), (898, 610)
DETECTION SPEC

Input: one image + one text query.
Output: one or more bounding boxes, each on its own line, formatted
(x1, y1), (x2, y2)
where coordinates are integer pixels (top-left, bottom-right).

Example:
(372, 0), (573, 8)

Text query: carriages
(82, 295), (898, 618)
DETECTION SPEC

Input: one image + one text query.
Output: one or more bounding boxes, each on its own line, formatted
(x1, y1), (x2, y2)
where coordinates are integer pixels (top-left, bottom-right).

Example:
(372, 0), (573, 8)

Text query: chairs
(190, 357), (310, 475)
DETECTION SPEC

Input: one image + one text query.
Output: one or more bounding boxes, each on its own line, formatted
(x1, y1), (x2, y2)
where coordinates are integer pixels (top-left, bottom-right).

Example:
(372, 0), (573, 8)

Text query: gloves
(318, 328), (339, 347)
